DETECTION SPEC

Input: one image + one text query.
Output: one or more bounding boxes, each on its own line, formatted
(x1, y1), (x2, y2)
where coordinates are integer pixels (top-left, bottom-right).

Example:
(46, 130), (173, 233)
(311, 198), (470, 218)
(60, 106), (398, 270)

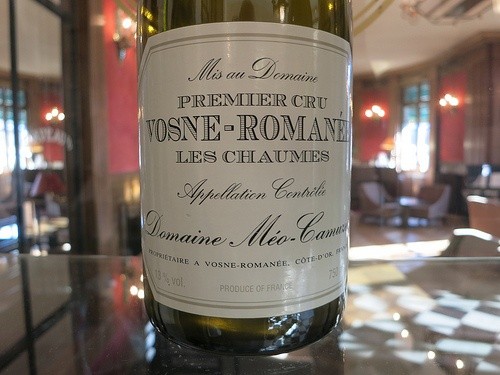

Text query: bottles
(137, 0), (354, 356)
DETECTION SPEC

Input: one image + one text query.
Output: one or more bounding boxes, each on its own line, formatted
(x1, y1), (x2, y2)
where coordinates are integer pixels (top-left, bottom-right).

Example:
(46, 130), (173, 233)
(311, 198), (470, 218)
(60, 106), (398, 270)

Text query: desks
(1, 251), (500, 375)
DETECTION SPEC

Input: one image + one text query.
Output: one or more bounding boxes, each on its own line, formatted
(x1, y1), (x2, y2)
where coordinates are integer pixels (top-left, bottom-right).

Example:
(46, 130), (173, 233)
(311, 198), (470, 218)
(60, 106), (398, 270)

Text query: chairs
(357, 182), (404, 226)
(410, 185), (451, 226)
